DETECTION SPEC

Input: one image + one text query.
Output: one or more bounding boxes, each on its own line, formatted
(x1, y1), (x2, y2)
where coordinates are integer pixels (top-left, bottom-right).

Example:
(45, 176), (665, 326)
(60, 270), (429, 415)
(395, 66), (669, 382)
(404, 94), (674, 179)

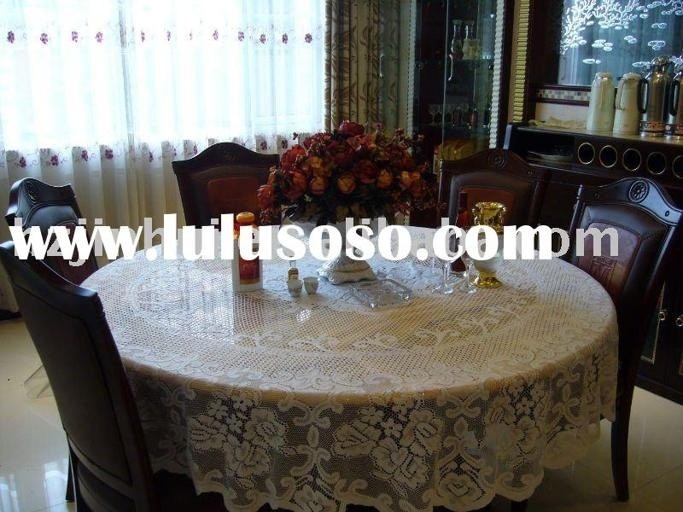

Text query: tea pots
(584, 56), (683, 137)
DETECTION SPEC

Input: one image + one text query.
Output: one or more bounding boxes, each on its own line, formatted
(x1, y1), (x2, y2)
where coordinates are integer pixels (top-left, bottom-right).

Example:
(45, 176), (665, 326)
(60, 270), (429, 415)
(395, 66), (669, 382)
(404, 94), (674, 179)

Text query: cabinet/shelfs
(502, 119), (683, 406)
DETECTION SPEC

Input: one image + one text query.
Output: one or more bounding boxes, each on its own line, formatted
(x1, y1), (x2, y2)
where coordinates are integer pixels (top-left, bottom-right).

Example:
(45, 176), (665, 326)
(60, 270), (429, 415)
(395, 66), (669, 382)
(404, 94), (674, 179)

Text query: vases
(303, 217), (383, 284)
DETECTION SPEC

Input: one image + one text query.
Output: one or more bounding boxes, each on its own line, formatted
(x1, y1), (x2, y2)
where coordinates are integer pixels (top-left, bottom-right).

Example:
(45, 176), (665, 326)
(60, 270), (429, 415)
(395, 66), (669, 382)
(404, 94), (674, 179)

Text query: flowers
(258, 120), (446, 227)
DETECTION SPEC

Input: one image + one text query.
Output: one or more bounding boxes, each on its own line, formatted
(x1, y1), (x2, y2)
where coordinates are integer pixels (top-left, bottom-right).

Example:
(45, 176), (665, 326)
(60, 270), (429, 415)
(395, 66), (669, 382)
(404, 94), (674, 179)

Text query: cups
(286, 276), (319, 297)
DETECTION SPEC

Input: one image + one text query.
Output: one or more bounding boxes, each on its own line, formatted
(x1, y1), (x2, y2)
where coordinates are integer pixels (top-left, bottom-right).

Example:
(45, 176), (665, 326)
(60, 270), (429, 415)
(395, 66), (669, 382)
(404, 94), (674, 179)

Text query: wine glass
(429, 103), (469, 125)
(432, 202), (507, 294)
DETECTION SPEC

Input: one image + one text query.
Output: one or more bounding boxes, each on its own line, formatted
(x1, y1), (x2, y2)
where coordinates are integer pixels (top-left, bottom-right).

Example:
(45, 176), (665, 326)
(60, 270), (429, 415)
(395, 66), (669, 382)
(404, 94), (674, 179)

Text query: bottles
(449, 20), (474, 59)
(288, 256), (298, 280)
(232, 212), (263, 292)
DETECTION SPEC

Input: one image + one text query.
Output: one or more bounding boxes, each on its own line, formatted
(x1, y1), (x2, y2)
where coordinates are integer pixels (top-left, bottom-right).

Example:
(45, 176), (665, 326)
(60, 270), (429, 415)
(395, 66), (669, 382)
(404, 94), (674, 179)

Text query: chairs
(172, 143), (280, 231)
(4, 177), (99, 285)
(558, 175), (683, 503)
(437, 146), (546, 239)
(0, 238), (265, 511)
